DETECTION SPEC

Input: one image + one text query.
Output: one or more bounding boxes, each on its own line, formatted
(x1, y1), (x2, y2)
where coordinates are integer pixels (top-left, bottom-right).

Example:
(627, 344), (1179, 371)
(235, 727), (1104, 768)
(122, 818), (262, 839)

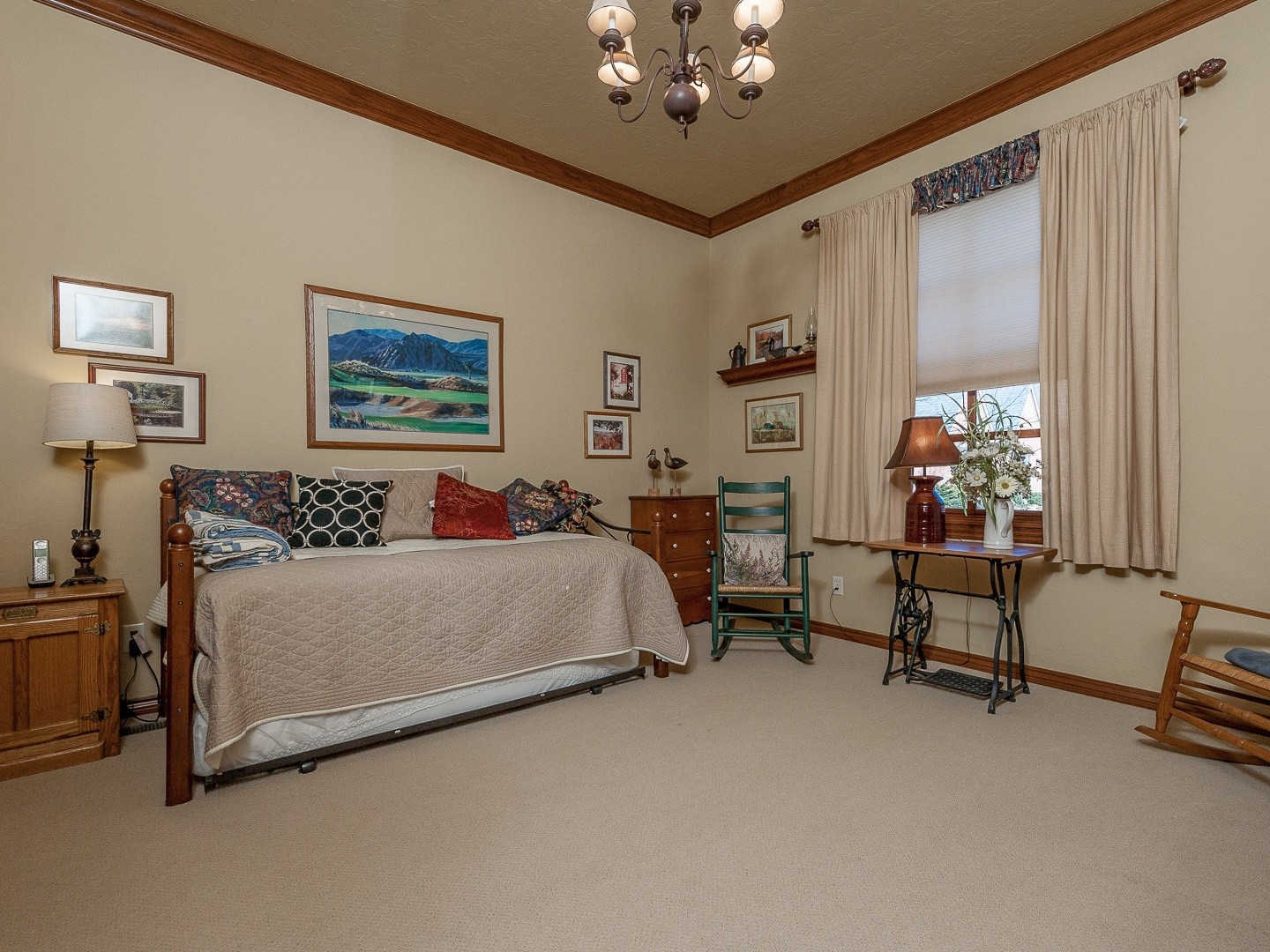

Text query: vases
(984, 497), (1015, 550)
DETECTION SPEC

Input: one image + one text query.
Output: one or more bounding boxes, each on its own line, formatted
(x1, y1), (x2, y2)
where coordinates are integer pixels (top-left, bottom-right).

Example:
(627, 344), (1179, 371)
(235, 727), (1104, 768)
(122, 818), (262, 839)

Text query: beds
(155, 478), (689, 805)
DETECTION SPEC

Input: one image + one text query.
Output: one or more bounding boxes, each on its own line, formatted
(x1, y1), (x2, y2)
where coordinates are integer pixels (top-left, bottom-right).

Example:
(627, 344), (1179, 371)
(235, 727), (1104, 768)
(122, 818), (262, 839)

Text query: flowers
(937, 385), (1046, 531)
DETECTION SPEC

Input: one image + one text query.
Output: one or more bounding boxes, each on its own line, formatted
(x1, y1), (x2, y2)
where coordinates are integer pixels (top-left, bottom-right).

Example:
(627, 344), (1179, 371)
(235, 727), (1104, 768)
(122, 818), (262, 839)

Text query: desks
(861, 539), (1058, 714)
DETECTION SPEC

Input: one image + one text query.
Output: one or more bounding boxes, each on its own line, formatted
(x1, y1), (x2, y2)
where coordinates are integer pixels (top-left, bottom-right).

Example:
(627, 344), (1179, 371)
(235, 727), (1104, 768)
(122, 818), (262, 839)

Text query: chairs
(1134, 590), (1270, 767)
(706, 475), (815, 661)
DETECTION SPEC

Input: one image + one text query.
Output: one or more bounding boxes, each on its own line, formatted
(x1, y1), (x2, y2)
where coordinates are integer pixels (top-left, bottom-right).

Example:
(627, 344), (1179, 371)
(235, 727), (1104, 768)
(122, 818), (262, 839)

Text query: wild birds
(647, 447), (689, 489)
(762, 337), (802, 362)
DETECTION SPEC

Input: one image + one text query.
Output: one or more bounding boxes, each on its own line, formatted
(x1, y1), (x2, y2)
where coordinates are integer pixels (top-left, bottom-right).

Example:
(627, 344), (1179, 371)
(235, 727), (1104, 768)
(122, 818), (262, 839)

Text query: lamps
(882, 415), (963, 543)
(42, 383), (137, 587)
(587, 0), (785, 140)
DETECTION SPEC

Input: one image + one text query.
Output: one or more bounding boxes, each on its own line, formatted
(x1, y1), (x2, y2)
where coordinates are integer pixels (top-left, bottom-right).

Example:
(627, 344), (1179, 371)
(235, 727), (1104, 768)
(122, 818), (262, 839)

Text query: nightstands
(0, 577), (128, 781)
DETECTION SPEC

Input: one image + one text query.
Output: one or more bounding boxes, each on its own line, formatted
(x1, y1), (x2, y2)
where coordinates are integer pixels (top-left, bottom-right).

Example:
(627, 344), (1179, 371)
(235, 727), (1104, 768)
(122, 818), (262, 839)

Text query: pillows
(170, 464), (604, 548)
(720, 534), (788, 586)
(1224, 648), (1270, 677)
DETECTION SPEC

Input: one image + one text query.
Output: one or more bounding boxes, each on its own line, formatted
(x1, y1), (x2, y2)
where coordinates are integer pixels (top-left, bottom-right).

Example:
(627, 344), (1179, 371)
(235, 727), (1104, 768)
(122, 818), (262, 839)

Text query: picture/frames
(51, 274), (206, 444)
(745, 315), (804, 454)
(305, 283), (504, 453)
(583, 351), (642, 459)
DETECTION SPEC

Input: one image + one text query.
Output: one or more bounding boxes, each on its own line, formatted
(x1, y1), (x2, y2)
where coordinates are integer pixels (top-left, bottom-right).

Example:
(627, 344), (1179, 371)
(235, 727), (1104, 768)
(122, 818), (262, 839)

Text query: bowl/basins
(769, 345), (803, 361)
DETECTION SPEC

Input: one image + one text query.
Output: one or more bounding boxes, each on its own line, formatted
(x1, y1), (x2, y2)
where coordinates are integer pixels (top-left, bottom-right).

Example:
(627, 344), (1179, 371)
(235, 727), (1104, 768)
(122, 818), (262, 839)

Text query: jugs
(729, 342), (747, 369)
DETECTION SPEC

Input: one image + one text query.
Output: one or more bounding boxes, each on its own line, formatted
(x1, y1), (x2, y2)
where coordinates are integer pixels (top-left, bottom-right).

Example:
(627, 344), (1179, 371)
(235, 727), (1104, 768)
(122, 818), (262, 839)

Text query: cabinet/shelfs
(629, 494), (720, 627)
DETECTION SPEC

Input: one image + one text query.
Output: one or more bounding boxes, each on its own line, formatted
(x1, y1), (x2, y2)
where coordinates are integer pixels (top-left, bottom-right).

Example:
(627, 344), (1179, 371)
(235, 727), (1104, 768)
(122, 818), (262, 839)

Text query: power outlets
(122, 623), (147, 653)
(832, 575), (844, 596)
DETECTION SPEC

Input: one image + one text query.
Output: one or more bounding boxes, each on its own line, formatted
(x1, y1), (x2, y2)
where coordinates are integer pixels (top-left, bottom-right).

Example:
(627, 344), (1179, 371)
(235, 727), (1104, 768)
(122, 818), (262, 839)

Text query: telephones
(27, 539), (55, 588)
